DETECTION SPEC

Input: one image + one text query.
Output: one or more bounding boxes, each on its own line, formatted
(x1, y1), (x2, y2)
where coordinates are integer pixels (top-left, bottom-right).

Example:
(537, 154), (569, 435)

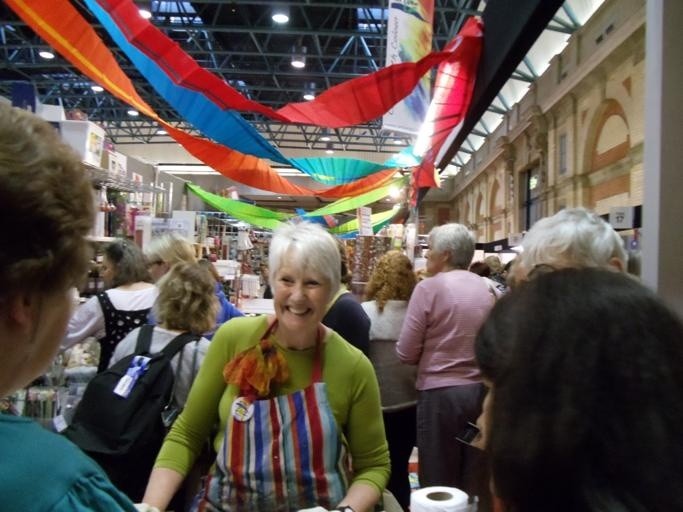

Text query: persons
(320, 207), (641, 511)
(58, 231), (273, 511)
(133, 220), (391, 511)
(472, 267), (683, 511)
(0, 93), (137, 511)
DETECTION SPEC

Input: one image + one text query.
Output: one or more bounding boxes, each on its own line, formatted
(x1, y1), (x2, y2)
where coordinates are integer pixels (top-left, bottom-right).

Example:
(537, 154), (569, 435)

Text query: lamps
(38, 43), (55, 59)
(271, 5), (290, 24)
(127, 110), (140, 116)
(291, 46), (307, 68)
(90, 85), (104, 92)
(321, 128), (330, 142)
(326, 142), (333, 154)
(303, 82), (316, 100)
(139, 4), (152, 19)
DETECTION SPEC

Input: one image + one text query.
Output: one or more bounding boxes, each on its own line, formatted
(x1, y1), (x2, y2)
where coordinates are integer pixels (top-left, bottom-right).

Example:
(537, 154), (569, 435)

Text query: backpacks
(59, 320), (198, 512)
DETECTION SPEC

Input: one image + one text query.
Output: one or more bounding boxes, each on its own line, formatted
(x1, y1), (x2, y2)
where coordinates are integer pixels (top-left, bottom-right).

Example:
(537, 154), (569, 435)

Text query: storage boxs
(102, 150), (117, 171)
(61, 120), (105, 167)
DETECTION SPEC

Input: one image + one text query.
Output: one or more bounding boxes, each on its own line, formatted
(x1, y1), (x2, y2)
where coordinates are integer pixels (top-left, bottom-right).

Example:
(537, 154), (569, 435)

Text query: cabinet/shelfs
(77, 162), (391, 297)
